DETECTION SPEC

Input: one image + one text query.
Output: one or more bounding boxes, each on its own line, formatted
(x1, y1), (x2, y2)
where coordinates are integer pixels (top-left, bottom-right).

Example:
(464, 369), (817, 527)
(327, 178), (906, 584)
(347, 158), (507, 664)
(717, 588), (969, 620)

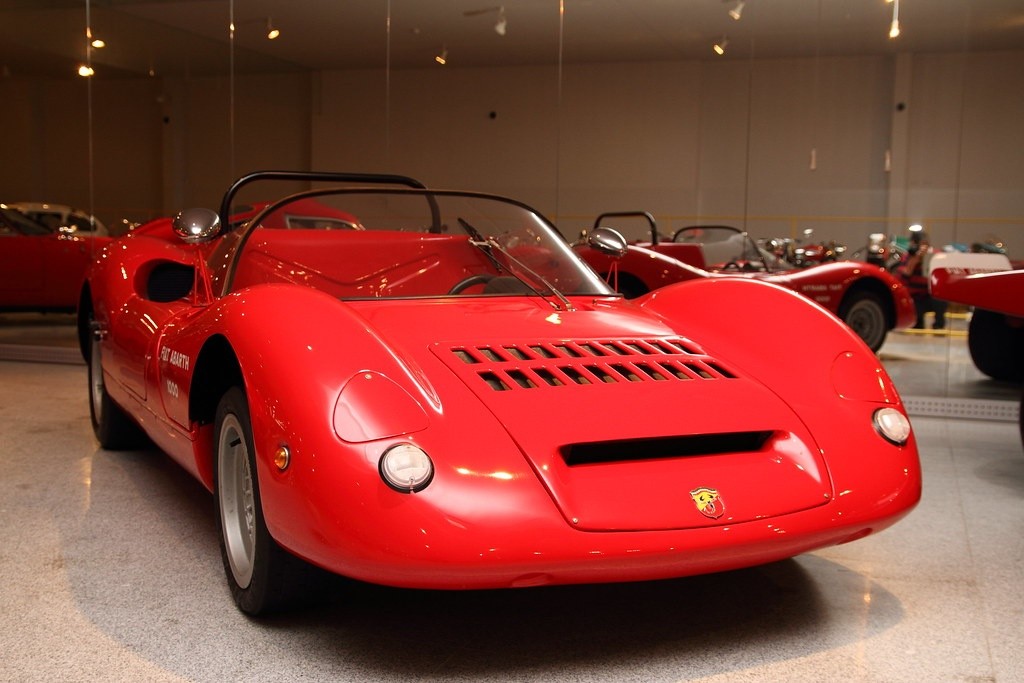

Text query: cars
(0, 201), (117, 310)
(229, 194), (365, 231)
(926, 250), (1024, 390)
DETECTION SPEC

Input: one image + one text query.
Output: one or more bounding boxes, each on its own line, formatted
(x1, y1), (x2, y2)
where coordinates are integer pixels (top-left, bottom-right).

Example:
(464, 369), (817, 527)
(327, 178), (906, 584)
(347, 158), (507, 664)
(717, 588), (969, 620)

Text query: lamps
(728, 0), (746, 20)
(435, 43), (450, 65)
(494, 6), (508, 36)
(713, 34), (730, 55)
(266, 18), (279, 40)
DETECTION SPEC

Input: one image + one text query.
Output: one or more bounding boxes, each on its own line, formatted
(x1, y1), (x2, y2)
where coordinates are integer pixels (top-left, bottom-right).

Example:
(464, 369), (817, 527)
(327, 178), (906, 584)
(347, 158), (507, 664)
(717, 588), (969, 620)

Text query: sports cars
(509, 208), (919, 355)
(75, 169), (925, 623)
